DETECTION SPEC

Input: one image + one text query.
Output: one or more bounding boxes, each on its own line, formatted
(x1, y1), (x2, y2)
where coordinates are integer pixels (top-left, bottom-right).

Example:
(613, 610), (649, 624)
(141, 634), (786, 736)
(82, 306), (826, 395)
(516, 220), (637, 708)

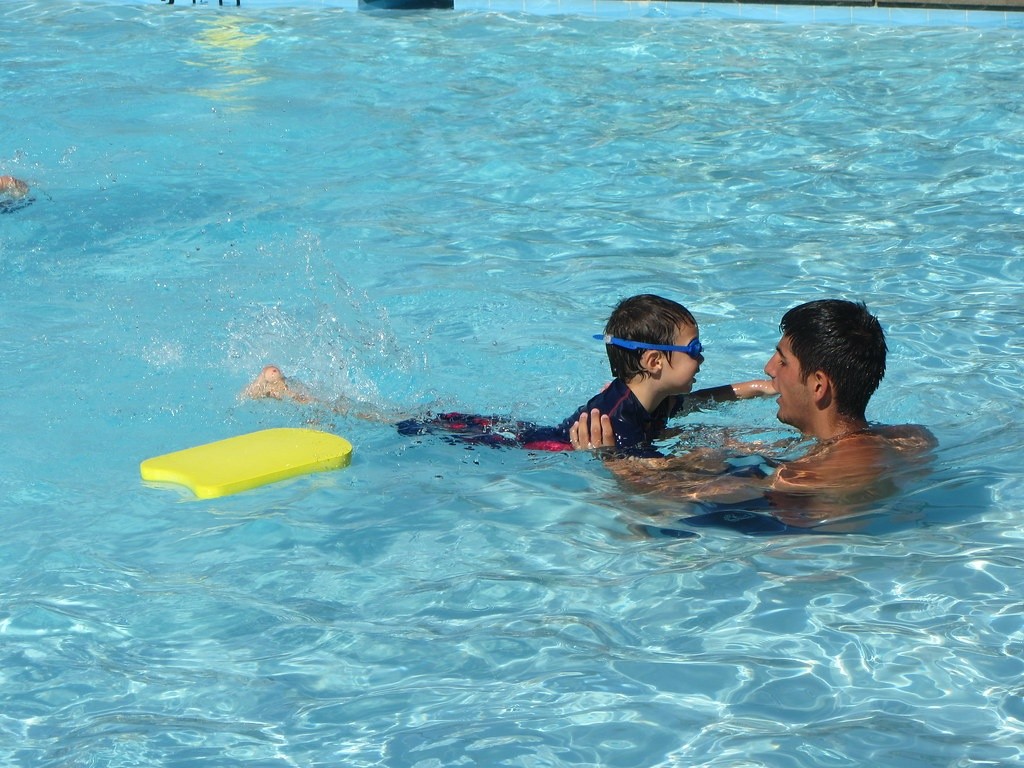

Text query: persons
(236, 294), (779, 471)
(0, 176), (29, 199)
(569, 297), (939, 534)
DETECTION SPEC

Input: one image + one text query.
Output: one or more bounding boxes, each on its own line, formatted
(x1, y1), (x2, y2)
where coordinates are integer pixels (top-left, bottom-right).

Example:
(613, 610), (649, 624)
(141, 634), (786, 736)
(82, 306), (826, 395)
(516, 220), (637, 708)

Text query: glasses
(686, 338), (702, 359)
